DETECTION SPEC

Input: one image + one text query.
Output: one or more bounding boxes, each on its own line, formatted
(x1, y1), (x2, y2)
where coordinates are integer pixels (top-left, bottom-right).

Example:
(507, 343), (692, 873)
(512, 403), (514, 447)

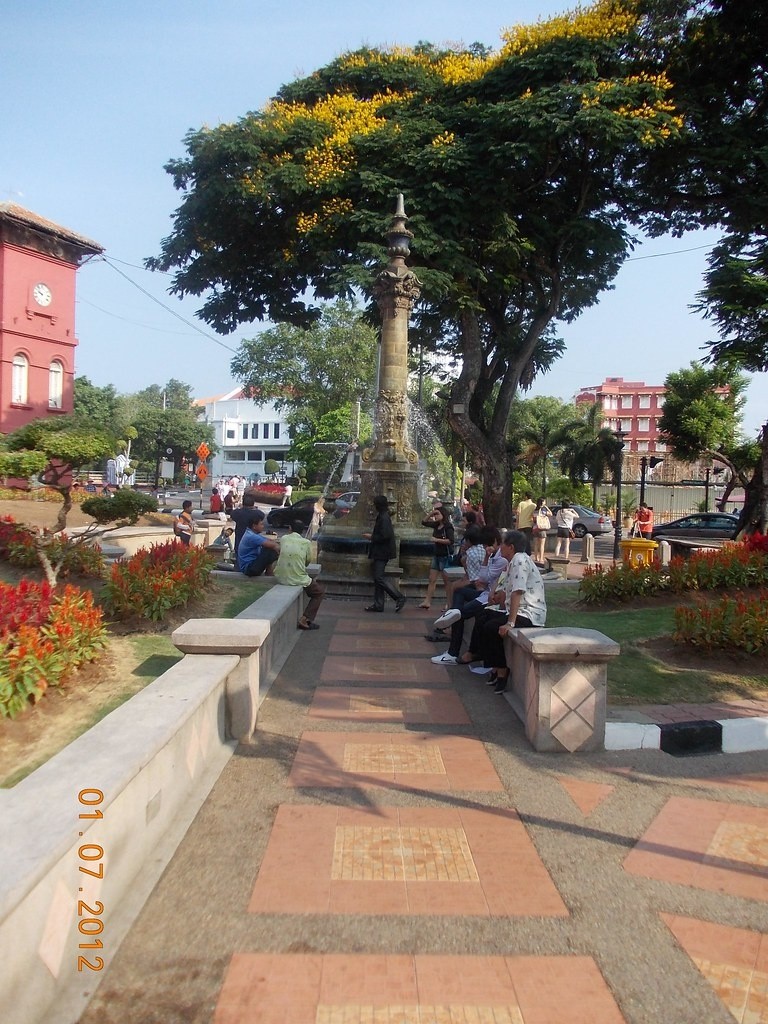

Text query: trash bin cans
(618, 537), (659, 569)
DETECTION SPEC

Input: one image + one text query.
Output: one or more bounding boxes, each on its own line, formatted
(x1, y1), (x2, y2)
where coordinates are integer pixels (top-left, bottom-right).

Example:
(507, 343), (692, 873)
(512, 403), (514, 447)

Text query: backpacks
(174, 512), (190, 536)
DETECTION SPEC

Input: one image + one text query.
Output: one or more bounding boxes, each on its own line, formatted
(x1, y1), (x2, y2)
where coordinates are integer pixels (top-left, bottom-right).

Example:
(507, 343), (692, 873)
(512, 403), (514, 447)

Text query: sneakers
(431, 651), (458, 666)
(434, 609), (461, 628)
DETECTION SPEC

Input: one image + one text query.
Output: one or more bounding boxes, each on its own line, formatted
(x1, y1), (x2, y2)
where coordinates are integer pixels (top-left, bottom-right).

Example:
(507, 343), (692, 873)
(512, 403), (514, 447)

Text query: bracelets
(507, 621), (514, 627)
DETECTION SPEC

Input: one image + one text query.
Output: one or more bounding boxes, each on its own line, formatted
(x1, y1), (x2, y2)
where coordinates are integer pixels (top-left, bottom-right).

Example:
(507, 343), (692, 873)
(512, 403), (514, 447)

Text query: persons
(237, 512), (280, 577)
(273, 519), (324, 629)
(466, 504), (486, 528)
(150, 485), (157, 497)
(177, 501), (193, 546)
(417, 507), (455, 612)
(84, 478), (97, 495)
(101, 482), (110, 495)
(218, 475), (246, 489)
(515, 492), (553, 562)
(280, 482), (292, 508)
(635, 503), (654, 539)
(555, 499), (579, 558)
(210, 488), (222, 513)
(231, 494), (265, 553)
(214, 526), (234, 549)
(73, 483), (79, 492)
(431, 525), (547, 694)
(184, 471), (196, 491)
(224, 491), (238, 515)
(362, 496), (406, 612)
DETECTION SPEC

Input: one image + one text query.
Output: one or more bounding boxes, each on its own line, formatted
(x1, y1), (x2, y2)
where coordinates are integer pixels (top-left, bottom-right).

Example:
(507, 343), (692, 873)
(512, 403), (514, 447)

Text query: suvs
(266, 499), (352, 531)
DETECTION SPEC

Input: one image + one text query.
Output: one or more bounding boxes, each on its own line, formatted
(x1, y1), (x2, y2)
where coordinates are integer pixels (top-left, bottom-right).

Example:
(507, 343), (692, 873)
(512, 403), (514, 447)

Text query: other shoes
(395, 596), (406, 611)
(487, 670), (498, 685)
(456, 651), (484, 664)
(364, 606), (384, 612)
(494, 667), (511, 693)
(298, 620), (319, 629)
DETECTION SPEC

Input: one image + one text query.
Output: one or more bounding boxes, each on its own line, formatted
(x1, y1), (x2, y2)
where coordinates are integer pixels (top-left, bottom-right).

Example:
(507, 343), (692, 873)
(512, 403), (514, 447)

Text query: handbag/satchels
(446, 554), (454, 565)
(536, 507), (551, 530)
(452, 554), (462, 566)
(568, 528), (576, 540)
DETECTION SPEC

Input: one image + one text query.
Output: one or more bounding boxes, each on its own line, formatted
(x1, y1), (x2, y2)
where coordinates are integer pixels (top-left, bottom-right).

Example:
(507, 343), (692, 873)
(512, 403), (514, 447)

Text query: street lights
(435, 391), (468, 507)
(290, 440), (297, 477)
(611, 427), (629, 559)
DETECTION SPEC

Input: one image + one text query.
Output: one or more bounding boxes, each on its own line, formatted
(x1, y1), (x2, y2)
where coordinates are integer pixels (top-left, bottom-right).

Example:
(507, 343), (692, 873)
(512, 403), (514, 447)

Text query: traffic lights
(650, 456), (666, 468)
(714, 468), (725, 474)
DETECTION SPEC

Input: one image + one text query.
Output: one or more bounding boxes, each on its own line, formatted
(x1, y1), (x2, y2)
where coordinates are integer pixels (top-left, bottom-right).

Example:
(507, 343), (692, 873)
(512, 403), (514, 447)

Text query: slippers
(415, 603), (430, 609)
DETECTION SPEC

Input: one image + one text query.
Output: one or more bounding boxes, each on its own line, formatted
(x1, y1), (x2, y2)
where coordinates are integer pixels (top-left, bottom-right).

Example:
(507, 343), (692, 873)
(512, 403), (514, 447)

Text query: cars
(632, 512), (738, 539)
(338, 492), (361, 506)
(537, 505), (614, 538)
